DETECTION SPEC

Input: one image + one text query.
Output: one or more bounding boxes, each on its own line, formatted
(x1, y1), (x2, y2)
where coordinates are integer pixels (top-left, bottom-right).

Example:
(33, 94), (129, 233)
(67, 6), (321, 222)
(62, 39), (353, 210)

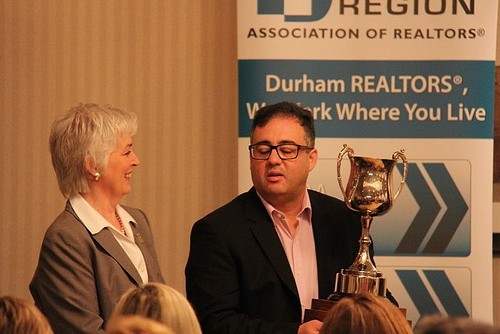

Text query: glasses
(249, 144), (313, 160)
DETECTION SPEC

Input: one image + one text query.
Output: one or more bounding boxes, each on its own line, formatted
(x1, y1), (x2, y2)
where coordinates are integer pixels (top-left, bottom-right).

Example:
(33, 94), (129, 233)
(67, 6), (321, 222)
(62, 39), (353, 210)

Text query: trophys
(303, 144), (412, 329)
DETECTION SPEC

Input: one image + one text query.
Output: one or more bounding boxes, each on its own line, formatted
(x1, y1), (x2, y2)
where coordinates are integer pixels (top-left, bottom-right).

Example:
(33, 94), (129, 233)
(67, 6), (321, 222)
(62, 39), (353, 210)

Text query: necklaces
(115, 212), (125, 234)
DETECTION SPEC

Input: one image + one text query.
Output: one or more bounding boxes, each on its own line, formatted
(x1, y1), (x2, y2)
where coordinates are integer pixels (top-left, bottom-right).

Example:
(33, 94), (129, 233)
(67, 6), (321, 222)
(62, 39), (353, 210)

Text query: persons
(29, 102), (167, 334)
(184, 101), (399, 334)
(106, 283), (500, 334)
(0, 295), (55, 334)
